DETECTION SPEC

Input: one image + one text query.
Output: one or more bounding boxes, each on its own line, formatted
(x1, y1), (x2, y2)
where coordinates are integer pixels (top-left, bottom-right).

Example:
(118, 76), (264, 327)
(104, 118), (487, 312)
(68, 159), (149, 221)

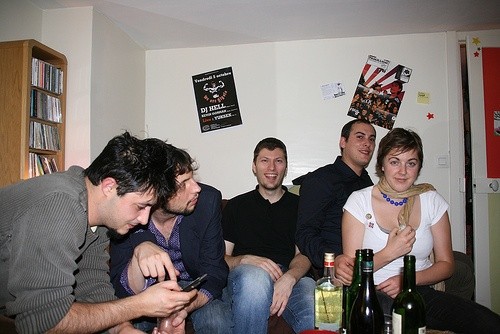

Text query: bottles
(391, 254), (427, 334)
(345, 249), (362, 329)
(315, 253), (343, 333)
(350, 248), (385, 334)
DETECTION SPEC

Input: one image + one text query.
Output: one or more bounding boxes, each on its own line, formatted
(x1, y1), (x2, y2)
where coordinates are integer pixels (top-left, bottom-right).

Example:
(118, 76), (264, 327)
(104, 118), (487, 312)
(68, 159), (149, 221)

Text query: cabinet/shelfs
(0, 39), (68, 189)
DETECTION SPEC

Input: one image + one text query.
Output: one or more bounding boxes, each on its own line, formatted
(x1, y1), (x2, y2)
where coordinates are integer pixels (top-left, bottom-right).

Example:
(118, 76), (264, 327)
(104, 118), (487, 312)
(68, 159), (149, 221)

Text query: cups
(383, 314), (392, 334)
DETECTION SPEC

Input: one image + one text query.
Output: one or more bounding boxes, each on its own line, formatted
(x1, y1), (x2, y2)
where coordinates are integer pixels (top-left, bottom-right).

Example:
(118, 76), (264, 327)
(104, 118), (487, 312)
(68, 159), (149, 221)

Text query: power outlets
(435, 154), (448, 167)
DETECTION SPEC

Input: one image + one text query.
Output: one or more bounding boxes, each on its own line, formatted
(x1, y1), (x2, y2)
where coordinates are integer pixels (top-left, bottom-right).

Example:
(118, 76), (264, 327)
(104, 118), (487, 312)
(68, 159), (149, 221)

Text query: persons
(110, 138), (233, 334)
(225, 137), (318, 334)
(345, 81), (402, 129)
(295, 122), (476, 296)
(342, 128), (500, 334)
(0, 132), (196, 334)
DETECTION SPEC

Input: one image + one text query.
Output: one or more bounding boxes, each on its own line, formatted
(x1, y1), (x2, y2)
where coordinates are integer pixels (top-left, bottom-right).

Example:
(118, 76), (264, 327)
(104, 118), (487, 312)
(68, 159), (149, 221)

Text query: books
(30, 90), (61, 124)
(29, 153), (60, 178)
(31, 58), (64, 95)
(29, 121), (60, 152)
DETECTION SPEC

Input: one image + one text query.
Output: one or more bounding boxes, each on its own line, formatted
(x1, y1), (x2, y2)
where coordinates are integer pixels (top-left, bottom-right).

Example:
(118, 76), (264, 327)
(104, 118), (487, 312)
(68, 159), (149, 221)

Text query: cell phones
(180, 274), (208, 292)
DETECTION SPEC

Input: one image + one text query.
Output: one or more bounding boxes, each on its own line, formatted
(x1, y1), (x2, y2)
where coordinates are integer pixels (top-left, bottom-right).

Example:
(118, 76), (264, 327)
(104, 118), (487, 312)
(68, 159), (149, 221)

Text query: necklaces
(381, 192), (407, 206)
